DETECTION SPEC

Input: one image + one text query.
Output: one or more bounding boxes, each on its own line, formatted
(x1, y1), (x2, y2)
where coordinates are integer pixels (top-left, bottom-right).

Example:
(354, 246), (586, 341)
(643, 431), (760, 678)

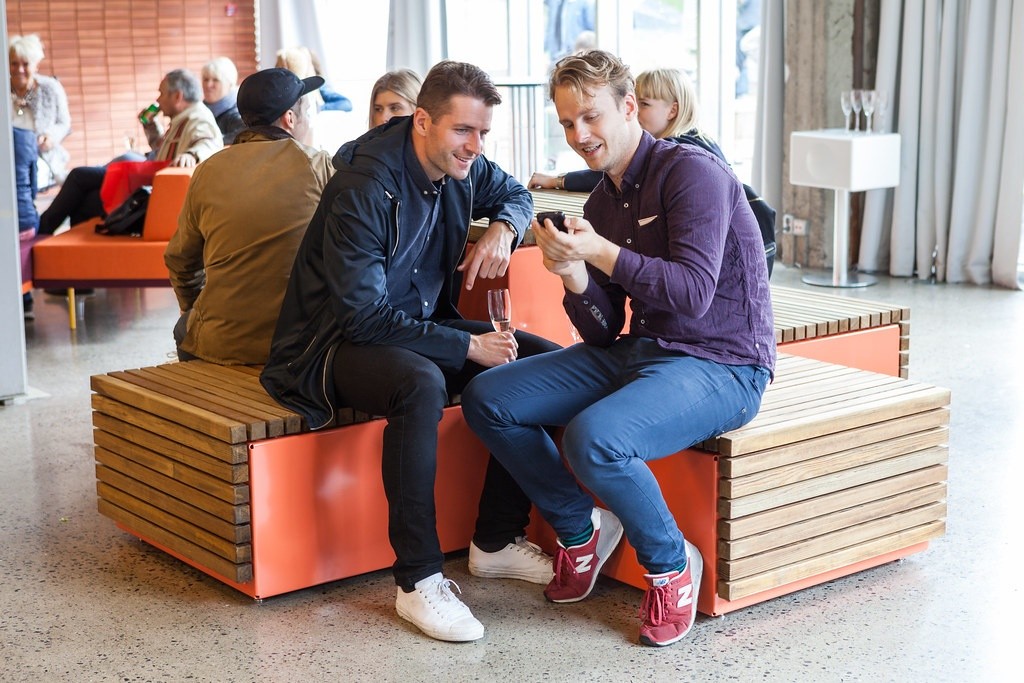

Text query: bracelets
(558, 172), (568, 189)
(499, 218), (517, 237)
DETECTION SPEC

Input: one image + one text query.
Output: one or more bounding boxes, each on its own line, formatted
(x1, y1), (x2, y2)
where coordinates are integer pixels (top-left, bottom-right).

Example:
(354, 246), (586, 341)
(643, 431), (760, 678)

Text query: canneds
(140, 103), (160, 124)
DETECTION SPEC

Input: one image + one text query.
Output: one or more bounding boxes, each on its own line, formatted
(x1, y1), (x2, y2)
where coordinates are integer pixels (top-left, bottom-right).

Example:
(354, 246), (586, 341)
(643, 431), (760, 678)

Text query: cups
(488, 289), (511, 334)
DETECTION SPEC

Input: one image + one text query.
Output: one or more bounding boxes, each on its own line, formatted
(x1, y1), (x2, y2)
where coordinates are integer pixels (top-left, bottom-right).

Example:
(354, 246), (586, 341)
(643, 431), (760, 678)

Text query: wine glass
(840, 90), (852, 136)
(851, 89), (863, 136)
(860, 90), (875, 137)
(874, 90), (887, 136)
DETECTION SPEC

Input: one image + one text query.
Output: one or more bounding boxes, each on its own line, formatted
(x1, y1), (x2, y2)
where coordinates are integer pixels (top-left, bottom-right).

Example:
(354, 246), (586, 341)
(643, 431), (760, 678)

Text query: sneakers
(640, 538), (704, 646)
(396, 572), (484, 641)
(543, 505), (624, 603)
(467, 535), (555, 584)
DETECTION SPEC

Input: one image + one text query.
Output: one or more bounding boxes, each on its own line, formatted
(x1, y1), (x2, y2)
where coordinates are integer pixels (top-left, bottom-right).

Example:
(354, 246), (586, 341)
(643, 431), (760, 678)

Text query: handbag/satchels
(94, 185), (153, 232)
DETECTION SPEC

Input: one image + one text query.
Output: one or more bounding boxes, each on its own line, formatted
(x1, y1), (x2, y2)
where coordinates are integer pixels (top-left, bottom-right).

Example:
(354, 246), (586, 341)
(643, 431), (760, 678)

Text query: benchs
(91, 284), (951, 616)
(31, 144), (231, 330)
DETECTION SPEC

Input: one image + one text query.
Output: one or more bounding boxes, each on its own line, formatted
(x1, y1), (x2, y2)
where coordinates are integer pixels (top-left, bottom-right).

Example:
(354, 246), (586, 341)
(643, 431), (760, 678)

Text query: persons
(12, 126), (39, 319)
(258, 57), (556, 642)
(306, 44), (353, 109)
(37, 67), (224, 300)
(9, 33), (71, 236)
(527, 66), (731, 192)
(369, 66), (425, 131)
(103, 56), (249, 168)
(276, 45), (324, 140)
(460, 47), (776, 646)
(163, 67), (338, 364)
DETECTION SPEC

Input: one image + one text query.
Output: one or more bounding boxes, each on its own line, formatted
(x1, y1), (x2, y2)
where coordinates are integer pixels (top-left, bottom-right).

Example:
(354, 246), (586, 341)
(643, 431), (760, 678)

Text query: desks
(456, 188), (632, 348)
(790, 129), (900, 287)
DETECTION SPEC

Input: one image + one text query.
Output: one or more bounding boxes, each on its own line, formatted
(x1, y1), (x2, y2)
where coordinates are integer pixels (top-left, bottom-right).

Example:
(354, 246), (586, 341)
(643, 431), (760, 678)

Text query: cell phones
(537, 211), (569, 233)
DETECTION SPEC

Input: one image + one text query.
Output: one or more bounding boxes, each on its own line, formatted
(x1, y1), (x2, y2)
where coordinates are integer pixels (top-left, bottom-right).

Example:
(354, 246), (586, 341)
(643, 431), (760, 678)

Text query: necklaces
(12, 87), (36, 115)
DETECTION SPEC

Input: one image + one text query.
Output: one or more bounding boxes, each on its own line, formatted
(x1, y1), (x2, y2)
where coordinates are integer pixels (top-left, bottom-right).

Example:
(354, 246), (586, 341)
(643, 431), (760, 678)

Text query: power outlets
(782, 215), (793, 235)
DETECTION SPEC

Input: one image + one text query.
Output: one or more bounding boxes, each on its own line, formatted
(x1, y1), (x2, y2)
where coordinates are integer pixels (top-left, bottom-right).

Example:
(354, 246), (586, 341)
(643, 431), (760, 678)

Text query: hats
(237, 67), (325, 126)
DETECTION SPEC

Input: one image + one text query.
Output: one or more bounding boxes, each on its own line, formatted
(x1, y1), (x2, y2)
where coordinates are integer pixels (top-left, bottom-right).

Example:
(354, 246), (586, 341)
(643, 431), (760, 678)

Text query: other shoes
(24, 292), (35, 318)
